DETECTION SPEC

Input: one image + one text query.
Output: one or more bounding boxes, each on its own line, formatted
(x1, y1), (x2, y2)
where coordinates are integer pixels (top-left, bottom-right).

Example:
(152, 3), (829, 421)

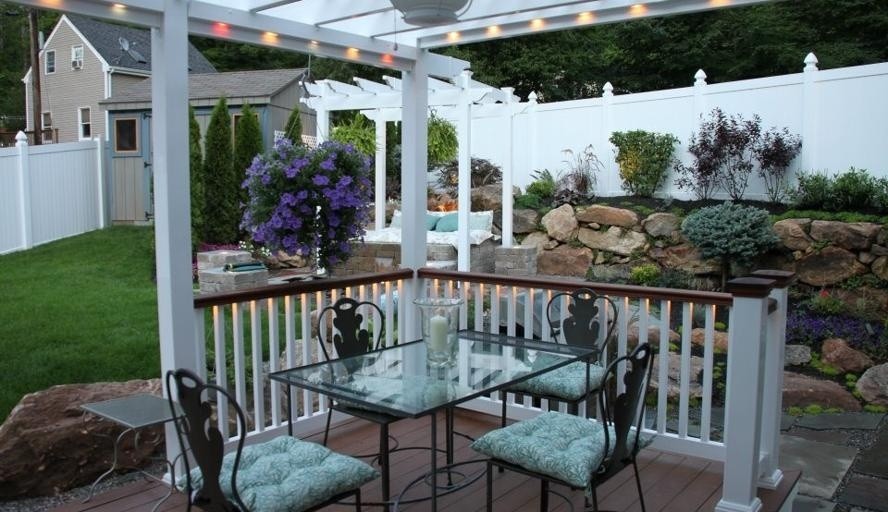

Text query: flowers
(235, 136), (373, 270)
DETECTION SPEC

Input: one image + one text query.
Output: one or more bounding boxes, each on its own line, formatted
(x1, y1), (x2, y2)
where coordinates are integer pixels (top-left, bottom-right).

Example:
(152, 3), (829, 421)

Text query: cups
(413, 298), (466, 369)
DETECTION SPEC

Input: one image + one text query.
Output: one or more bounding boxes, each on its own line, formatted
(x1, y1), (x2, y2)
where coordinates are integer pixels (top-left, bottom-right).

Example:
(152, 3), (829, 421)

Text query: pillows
(425, 212), (459, 232)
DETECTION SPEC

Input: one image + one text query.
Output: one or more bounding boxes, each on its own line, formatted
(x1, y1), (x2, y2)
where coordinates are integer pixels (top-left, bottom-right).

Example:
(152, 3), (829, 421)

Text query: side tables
(77, 392), (194, 511)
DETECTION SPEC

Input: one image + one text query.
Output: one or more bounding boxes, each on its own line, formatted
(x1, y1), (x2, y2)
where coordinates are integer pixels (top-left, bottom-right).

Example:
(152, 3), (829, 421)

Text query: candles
(412, 293), (465, 375)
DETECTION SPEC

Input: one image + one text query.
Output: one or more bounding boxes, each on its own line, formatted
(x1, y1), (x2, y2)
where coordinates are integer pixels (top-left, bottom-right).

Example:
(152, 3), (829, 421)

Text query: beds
(346, 208), (494, 258)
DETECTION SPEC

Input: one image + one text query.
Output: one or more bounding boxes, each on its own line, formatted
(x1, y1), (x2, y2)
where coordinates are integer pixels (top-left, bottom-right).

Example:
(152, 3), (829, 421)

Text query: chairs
(467, 342), (664, 511)
(499, 288), (617, 476)
(317, 296), (387, 468)
(165, 366), (382, 512)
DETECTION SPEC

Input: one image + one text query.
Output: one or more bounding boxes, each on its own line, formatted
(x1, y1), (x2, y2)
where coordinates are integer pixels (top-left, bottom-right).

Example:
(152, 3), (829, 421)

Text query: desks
(265, 332), (603, 512)
